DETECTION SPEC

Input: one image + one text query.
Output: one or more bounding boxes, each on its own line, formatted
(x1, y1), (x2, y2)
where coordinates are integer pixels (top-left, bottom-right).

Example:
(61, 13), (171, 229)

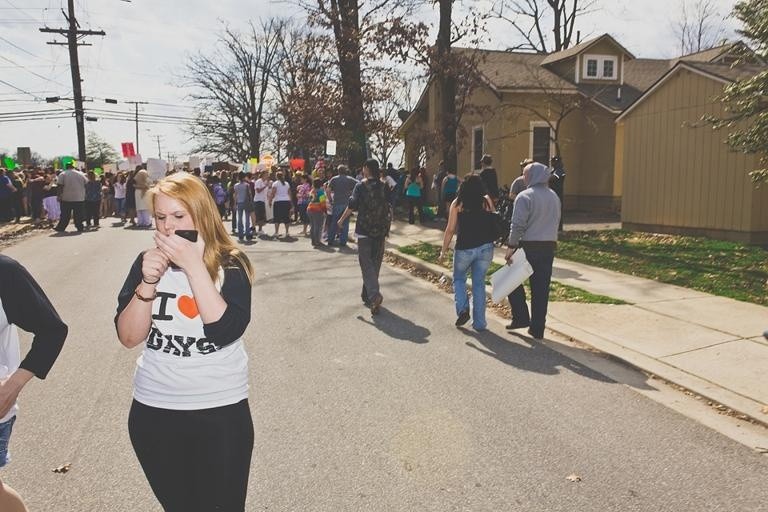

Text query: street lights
(45, 94), (117, 163)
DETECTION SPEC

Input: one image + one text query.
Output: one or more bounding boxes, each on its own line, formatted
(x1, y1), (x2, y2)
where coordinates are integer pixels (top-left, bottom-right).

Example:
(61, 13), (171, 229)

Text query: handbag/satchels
(466, 197), (504, 247)
(245, 184), (254, 212)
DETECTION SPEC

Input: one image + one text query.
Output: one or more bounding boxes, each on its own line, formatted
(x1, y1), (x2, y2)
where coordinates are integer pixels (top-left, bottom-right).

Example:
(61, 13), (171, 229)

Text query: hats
(480, 154), (493, 163)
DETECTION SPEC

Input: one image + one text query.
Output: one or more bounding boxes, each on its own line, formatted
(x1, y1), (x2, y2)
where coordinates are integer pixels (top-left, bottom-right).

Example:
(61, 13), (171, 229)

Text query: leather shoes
(528, 330), (544, 339)
(505, 321), (529, 329)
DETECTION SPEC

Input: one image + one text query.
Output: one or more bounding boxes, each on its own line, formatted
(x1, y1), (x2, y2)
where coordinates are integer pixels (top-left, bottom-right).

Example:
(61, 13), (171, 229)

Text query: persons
(0, 250), (72, 512)
(505, 156), (563, 343)
(502, 156), (560, 240)
(0, 153), (430, 250)
(432, 154), (500, 224)
(113, 170), (260, 511)
(337, 158), (395, 316)
(438, 172), (504, 334)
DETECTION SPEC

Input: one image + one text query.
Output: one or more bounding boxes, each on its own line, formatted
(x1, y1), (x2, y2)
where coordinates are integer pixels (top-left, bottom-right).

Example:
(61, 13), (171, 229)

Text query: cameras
(439, 277), (446, 285)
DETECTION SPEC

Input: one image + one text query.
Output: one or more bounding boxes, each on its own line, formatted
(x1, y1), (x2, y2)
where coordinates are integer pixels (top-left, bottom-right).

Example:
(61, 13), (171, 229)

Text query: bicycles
(491, 183), (523, 249)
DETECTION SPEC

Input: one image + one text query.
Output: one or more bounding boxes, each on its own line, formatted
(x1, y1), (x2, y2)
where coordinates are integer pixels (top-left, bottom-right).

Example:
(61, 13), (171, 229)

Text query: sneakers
(371, 294), (383, 316)
(455, 313), (470, 326)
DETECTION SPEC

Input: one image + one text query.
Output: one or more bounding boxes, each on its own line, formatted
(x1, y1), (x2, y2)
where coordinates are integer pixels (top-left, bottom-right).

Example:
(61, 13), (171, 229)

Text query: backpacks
(358, 177), (392, 240)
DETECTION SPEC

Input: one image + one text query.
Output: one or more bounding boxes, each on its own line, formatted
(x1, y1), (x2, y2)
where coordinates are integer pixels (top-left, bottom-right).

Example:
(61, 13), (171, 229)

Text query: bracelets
(142, 276), (162, 285)
(135, 288), (158, 302)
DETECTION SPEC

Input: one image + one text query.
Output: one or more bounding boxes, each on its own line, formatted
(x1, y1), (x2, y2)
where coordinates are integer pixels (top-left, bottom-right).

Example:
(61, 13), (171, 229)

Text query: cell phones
(170, 230), (199, 270)
